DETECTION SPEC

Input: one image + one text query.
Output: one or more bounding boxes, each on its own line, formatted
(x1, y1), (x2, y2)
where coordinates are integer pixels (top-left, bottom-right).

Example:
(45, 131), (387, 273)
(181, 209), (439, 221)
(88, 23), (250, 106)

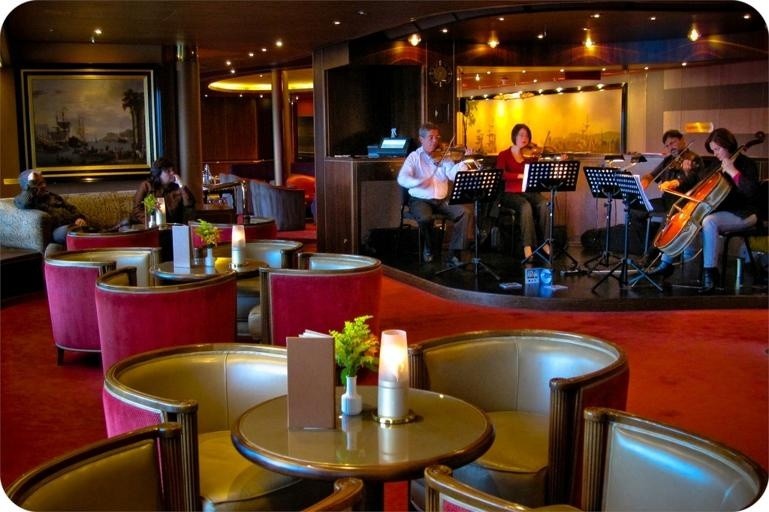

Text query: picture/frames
(13, 61), (168, 185)
(460, 81), (628, 161)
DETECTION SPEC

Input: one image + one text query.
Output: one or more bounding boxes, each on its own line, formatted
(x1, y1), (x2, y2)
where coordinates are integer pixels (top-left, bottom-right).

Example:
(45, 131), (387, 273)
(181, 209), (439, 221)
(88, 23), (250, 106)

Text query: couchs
(2, 188), (154, 294)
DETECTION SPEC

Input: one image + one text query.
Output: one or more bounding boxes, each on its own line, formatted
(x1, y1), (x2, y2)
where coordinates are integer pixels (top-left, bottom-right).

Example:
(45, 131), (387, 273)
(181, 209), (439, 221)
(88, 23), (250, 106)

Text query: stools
(645, 211), (668, 259)
(713, 181), (767, 294)
(398, 185), (446, 269)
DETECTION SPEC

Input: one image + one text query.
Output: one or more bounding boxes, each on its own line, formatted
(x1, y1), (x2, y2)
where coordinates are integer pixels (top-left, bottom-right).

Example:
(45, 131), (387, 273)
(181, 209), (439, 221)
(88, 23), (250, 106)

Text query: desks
(231, 383), (497, 511)
(148, 255), (269, 282)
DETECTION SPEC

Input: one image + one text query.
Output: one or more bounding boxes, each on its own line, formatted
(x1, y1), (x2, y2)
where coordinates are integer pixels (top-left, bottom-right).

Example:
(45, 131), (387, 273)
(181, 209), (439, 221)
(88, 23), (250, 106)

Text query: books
(283, 328), (337, 430)
(171, 222), (192, 275)
(143, 197), (168, 229)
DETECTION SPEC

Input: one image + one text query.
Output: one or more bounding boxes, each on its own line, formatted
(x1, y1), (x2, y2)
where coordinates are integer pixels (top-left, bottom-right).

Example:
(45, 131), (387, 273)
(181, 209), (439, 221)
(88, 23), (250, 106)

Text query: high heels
(697, 267), (719, 294)
(643, 262), (674, 283)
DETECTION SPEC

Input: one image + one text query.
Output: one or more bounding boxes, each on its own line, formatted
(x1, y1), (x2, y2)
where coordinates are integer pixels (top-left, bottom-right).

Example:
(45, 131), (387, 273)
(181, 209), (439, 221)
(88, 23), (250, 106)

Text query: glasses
(421, 135), (441, 141)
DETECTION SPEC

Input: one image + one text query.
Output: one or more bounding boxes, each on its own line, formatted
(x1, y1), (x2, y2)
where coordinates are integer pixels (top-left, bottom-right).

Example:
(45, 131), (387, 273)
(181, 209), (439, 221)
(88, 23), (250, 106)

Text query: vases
(340, 377), (362, 415)
(204, 248), (216, 274)
(148, 215), (156, 229)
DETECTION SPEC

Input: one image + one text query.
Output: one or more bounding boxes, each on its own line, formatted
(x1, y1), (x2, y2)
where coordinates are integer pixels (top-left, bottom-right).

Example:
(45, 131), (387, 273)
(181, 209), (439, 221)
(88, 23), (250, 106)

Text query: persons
(395, 121), (480, 268)
(648, 127), (761, 296)
(13, 168), (132, 246)
(133, 154), (196, 224)
(622, 127), (708, 265)
(495, 121), (558, 265)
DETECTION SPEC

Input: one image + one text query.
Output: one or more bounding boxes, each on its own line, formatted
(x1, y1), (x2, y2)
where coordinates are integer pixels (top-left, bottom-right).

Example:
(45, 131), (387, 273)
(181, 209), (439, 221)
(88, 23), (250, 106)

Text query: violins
(430, 143), (482, 162)
(520, 144), (572, 162)
(667, 152), (699, 171)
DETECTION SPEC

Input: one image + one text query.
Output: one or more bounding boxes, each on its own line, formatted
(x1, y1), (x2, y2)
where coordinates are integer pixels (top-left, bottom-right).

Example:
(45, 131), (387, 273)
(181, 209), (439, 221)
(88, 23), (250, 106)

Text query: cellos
(653, 132), (765, 257)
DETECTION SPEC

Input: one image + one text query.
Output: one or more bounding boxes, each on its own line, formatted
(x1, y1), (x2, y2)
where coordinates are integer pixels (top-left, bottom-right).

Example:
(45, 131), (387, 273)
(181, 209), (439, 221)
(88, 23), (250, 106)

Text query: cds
(540, 269), (552, 284)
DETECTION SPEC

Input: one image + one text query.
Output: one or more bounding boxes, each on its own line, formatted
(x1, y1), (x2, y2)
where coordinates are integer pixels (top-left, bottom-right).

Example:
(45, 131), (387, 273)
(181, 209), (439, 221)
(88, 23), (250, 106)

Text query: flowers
(195, 217), (221, 248)
(324, 314), (382, 376)
(141, 194), (163, 214)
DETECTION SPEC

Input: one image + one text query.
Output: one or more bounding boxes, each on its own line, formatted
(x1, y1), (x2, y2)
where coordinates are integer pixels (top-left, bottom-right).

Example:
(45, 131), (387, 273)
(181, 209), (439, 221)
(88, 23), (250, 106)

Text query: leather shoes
(448, 255), (468, 268)
(423, 241), (434, 265)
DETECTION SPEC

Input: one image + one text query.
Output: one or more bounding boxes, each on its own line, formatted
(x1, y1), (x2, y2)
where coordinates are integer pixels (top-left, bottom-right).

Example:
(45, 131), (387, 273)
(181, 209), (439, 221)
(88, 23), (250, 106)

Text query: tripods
(591, 201), (665, 291)
(583, 197), (623, 274)
(521, 190), (577, 269)
(434, 200), (502, 280)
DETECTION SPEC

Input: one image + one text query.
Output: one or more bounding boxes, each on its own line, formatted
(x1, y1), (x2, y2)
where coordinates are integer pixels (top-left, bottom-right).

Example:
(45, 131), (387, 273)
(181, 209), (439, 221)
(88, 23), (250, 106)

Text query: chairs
(66, 223), (159, 254)
(249, 178), (305, 230)
(249, 251), (383, 376)
(5, 422), (365, 508)
(94, 266), (238, 379)
(199, 239), (303, 340)
(188, 215), (279, 254)
(286, 174), (316, 202)
(99, 341), (325, 510)
(423, 406), (768, 510)
(407, 328), (631, 510)
